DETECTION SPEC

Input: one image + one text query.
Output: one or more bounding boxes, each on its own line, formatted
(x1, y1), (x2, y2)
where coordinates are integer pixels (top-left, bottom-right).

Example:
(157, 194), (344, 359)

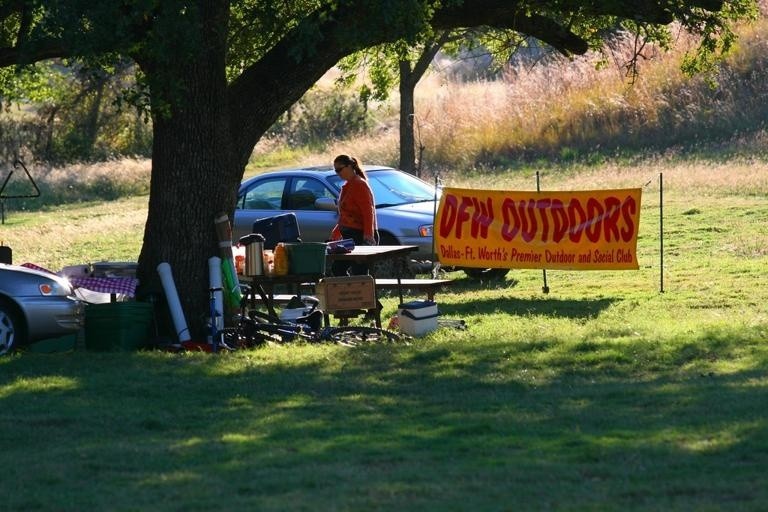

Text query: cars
(233, 162), (519, 283)
(1, 262), (87, 355)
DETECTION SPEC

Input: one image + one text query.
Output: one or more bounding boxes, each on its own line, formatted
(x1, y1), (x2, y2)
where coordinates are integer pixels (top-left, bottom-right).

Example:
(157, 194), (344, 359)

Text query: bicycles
(219, 308), (407, 350)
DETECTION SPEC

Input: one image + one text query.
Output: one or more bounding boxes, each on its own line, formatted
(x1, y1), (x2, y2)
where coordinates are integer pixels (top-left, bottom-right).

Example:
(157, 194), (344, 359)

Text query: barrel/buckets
(85, 301), (155, 353)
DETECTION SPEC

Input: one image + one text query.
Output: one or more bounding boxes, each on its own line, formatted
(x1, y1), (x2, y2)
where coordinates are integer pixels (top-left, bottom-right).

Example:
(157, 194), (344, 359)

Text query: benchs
(234, 239), (454, 327)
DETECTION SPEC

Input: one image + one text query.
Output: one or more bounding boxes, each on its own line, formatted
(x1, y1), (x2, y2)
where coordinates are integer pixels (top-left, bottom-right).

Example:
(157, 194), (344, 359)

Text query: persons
(327, 153), (384, 322)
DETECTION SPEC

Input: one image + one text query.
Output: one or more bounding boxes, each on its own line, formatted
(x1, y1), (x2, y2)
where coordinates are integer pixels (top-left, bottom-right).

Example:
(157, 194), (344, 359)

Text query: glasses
(334, 166), (346, 171)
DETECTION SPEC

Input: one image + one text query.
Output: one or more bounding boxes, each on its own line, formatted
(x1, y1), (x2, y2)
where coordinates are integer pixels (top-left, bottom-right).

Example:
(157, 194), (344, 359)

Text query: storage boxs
(83, 301), (158, 352)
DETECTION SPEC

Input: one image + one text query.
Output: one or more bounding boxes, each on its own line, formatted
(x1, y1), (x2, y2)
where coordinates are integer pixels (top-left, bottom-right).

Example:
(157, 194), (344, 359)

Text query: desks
(71, 276), (137, 304)
